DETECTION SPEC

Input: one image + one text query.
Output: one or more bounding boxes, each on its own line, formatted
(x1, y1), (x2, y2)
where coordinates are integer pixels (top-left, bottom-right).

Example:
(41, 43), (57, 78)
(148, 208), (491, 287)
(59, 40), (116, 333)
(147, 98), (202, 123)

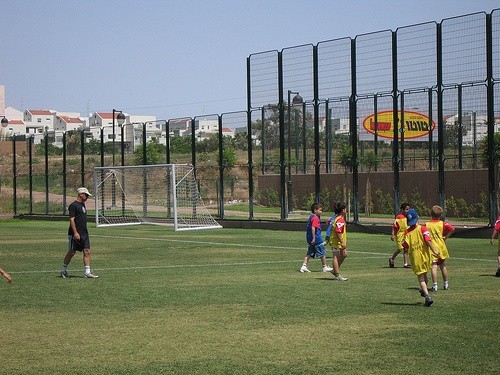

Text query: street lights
(288, 90), (303, 214)
(106, 109), (126, 209)
(0, 116), (8, 127)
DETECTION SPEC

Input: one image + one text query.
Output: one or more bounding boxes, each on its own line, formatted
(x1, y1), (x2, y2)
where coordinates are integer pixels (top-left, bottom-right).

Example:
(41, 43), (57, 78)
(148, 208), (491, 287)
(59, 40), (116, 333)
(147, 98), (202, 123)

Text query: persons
(490, 203), (500, 277)
(329, 202), (348, 281)
(60, 187), (99, 279)
(421, 205), (455, 292)
(322, 212), (339, 246)
(299, 203), (334, 273)
(402, 208), (440, 307)
(389, 203), (412, 268)
(0, 268), (12, 284)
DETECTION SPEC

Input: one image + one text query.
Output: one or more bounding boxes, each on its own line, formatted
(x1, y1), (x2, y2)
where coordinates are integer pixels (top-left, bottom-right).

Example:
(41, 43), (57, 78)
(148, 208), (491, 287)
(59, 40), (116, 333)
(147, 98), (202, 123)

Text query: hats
(77, 187), (92, 196)
(405, 210), (418, 226)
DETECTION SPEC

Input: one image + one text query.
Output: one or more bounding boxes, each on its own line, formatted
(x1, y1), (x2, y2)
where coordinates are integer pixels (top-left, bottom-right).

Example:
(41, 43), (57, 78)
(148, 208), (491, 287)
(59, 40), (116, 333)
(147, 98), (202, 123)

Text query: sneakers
(84, 272), (98, 278)
(419, 288), (425, 298)
(389, 258), (394, 268)
(403, 264), (412, 268)
(61, 269), (68, 278)
(299, 266), (311, 272)
(495, 267), (500, 277)
(335, 276), (348, 281)
(330, 269), (334, 275)
(430, 286), (437, 292)
(424, 297), (434, 307)
(444, 283), (448, 289)
(322, 265), (333, 272)
